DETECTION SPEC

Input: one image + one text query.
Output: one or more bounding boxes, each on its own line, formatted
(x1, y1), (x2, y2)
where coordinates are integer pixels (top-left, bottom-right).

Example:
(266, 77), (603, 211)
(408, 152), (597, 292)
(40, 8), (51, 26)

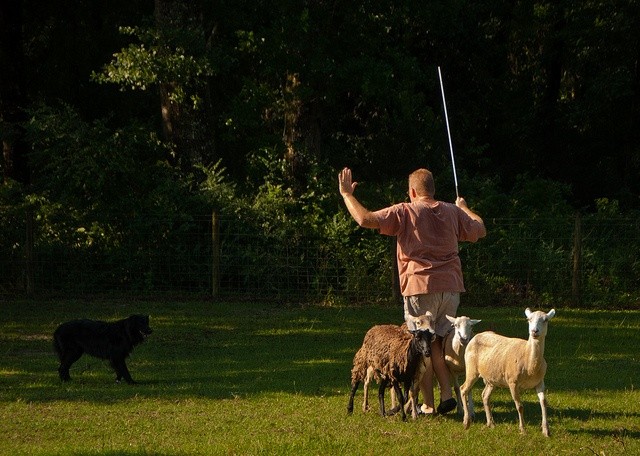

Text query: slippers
(436, 398), (457, 414)
(415, 403), (433, 415)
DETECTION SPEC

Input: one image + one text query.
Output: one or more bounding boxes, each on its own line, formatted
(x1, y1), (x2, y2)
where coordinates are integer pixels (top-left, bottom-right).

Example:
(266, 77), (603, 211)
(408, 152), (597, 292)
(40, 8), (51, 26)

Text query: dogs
(52, 314), (152, 384)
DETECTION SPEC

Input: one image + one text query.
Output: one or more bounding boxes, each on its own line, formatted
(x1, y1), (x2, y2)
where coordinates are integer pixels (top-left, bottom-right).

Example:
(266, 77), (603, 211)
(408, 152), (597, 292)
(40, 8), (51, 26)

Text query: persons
(338, 167), (488, 416)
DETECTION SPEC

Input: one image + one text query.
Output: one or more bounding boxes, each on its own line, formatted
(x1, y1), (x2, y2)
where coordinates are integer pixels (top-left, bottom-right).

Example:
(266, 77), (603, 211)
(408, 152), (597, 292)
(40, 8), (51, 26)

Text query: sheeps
(461, 306), (556, 438)
(363, 311), (441, 420)
(438, 310), (482, 418)
(347, 325), (433, 420)
